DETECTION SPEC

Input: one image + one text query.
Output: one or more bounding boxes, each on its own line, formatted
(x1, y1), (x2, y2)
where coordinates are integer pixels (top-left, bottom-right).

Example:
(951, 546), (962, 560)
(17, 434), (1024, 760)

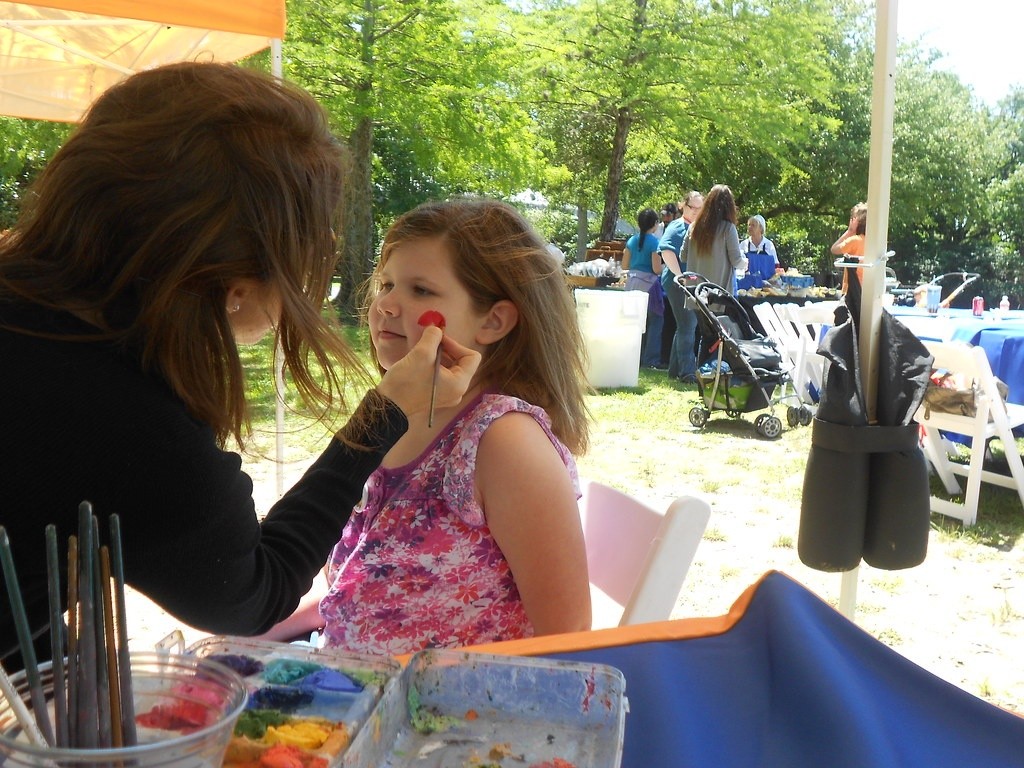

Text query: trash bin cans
(574, 288), (649, 388)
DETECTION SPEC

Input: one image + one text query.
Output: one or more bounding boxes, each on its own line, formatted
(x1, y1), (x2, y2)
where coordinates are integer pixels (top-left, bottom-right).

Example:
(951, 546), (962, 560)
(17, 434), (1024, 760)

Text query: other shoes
(680, 376), (698, 383)
(646, 362), (669, 371)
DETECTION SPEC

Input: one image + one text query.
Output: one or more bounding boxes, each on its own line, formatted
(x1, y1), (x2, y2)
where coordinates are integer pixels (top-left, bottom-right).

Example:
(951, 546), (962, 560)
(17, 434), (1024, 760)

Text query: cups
(1, 650), (248, 767)
(926, 285), (943, 314)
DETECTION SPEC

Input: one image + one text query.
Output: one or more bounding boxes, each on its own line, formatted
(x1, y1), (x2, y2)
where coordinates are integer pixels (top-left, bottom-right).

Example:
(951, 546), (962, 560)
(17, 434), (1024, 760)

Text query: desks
(819, 305), (1024, 463)
(736, 295), (839, 383)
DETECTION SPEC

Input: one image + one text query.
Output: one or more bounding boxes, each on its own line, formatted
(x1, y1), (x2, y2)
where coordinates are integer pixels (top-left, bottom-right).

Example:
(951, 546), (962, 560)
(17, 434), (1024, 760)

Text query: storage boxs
(152, 630), (631, 768)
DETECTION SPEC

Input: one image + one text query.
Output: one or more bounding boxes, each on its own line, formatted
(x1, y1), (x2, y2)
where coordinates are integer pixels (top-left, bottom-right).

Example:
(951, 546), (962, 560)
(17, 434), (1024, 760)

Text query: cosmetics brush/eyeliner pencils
(428, 321), (444, 428)
(1, 501), (138, 767)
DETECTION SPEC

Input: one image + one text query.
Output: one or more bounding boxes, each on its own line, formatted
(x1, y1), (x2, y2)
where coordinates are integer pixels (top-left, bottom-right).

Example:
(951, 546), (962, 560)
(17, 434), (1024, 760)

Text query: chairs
(752, 302), (840, 415)
(913, 338), (1024, 528)
(578, 472), (712, 629)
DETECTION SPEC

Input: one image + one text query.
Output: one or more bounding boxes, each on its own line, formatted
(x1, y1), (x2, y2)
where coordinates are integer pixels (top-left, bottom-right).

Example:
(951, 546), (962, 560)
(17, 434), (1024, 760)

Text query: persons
(679, 185), (748, 396)
(251, 196), (587, 655)
(0, 63), (483, 708)
(622, 209), (669, 372)
(738, 214), (781, 291)
(654, 203), (676, 237)
(661, 192), (704, 382)
(831, 201), (867, 295)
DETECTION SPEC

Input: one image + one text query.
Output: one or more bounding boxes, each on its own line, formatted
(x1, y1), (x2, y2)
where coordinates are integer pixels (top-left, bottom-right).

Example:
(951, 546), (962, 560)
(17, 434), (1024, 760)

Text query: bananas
(815, 287), (825, 297)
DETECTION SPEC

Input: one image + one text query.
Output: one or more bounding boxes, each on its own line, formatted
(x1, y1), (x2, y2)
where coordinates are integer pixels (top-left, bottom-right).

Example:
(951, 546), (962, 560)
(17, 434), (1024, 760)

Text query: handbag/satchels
(921, 367), (1010, 421)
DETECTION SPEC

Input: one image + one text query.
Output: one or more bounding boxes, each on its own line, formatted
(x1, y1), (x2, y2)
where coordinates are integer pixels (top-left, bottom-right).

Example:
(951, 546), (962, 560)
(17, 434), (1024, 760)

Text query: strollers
(888, 272), (981, 308)
(674, 271), (812, 439)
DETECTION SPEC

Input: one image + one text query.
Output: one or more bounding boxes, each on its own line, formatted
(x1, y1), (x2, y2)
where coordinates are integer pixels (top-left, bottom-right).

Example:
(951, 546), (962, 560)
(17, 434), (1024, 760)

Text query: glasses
(686, 204), (702, 210)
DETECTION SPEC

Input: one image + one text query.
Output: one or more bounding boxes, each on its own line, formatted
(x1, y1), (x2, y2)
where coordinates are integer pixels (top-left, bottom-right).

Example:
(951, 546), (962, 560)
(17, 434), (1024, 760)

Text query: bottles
(1000, 296), (1010, 312)
(735, 250), (746, 279)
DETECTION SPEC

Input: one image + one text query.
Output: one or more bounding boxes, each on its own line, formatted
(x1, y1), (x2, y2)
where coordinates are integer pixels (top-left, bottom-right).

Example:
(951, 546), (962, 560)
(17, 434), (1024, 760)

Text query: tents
(0, 0), (287, 123)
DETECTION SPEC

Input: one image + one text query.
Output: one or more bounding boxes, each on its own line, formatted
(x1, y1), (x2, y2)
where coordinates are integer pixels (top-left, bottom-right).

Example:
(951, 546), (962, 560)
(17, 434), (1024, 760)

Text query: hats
(660, 203), (675, 215)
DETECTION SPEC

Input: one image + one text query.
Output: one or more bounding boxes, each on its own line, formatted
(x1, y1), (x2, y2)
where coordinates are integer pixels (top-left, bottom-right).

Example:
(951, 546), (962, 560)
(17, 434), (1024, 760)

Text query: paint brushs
(47, 525), (71, 748)
(428, 323), (445, 428)
(66, 501), (140, 767)
(0, 661), (60, 768)
(0, 526), (55, 748)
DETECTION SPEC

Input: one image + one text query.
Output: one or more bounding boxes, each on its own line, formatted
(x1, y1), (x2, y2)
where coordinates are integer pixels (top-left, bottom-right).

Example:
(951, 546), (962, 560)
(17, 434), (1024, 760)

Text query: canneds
(973, 296), (984, 316)
